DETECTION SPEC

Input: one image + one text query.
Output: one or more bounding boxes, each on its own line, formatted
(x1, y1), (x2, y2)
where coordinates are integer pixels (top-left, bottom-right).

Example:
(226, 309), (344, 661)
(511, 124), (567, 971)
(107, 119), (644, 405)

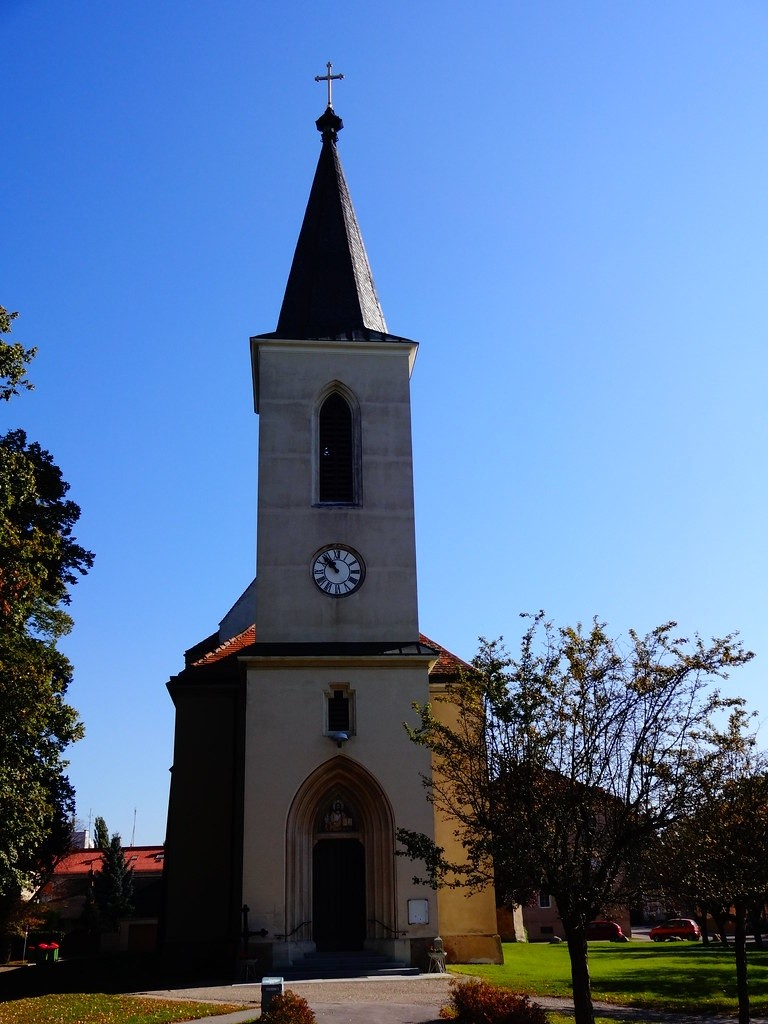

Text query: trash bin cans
(260, 976), (285, 1019)
(27, 942), (58, 963)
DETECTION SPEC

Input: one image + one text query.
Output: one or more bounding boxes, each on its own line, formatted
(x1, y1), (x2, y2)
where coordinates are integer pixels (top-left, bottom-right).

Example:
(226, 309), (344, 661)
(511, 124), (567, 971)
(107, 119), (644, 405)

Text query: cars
(584, 920), (621, 942)
(649, 918), (701, 943)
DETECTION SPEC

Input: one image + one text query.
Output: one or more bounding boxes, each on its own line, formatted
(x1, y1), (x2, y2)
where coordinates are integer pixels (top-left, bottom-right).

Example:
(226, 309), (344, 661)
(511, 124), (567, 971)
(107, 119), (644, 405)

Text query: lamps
(333, 732), (348, 748)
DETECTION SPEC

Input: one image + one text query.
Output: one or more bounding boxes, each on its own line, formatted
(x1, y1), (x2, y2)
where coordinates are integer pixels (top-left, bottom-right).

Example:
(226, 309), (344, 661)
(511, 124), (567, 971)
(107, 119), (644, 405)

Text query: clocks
(309, 543), (366, 598)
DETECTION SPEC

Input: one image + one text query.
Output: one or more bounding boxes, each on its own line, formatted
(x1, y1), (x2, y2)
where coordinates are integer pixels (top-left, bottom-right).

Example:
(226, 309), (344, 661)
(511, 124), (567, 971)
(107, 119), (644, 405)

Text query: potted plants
(427, 946), (447, 958)
